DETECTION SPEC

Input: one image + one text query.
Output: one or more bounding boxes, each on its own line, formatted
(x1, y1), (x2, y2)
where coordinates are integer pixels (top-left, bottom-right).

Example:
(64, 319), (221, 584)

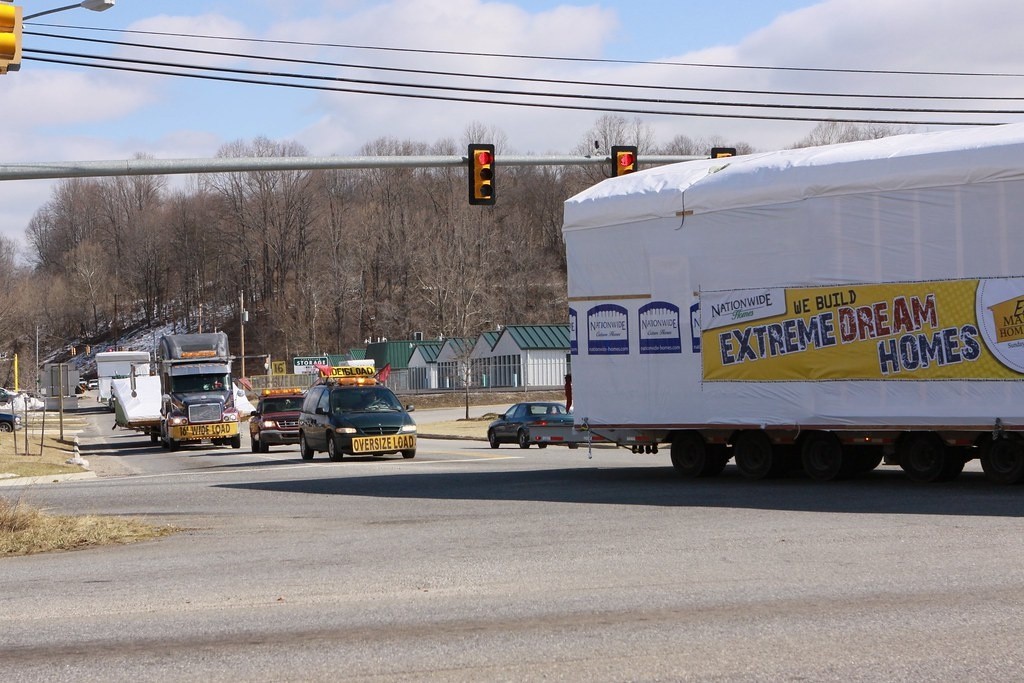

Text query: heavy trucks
(563, 119), (1024, 481)
(144, 333), (242, 450)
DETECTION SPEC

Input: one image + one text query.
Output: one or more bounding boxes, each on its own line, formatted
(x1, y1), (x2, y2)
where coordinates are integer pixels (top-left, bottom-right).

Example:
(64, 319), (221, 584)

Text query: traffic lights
(467, 142), (496, 205)
(609, 145), (639, 175)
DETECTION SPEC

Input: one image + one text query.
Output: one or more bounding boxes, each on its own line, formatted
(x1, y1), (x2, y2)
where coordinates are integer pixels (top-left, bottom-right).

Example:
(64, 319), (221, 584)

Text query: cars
(488, 402), (594, 450)
(249, 388), (312, 453)
(297, 366), (420, 460)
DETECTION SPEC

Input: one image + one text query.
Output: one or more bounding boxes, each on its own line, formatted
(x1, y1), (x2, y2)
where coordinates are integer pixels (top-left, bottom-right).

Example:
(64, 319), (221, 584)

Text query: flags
(313, 363), (334, 378)
(239, 378), (252, 389)
(379, 364), (390, 382)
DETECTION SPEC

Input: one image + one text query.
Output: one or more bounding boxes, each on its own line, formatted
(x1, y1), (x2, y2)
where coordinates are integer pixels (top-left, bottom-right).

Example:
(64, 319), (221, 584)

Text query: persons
(367, 393), (389, 409)
(212, 378), (222, 390)
